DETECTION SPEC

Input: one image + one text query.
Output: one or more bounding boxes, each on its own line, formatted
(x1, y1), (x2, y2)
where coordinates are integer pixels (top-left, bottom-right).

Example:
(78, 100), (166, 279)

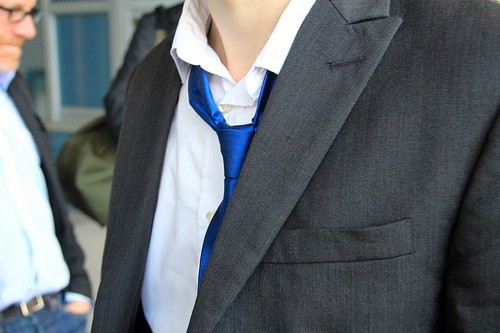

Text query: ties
(188, 66), (278, 295)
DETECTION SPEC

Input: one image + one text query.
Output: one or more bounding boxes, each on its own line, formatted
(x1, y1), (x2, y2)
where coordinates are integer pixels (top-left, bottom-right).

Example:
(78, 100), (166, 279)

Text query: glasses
(1, 6), (42, 24)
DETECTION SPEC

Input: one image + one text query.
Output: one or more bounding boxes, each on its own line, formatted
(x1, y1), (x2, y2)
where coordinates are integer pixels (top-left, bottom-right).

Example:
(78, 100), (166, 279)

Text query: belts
(0, 294), (54, 321)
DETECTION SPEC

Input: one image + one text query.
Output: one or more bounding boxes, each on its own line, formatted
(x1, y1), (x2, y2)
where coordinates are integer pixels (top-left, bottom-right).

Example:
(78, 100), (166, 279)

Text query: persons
(0, 0), (94, 333)
(91, 0), (500, 333)
